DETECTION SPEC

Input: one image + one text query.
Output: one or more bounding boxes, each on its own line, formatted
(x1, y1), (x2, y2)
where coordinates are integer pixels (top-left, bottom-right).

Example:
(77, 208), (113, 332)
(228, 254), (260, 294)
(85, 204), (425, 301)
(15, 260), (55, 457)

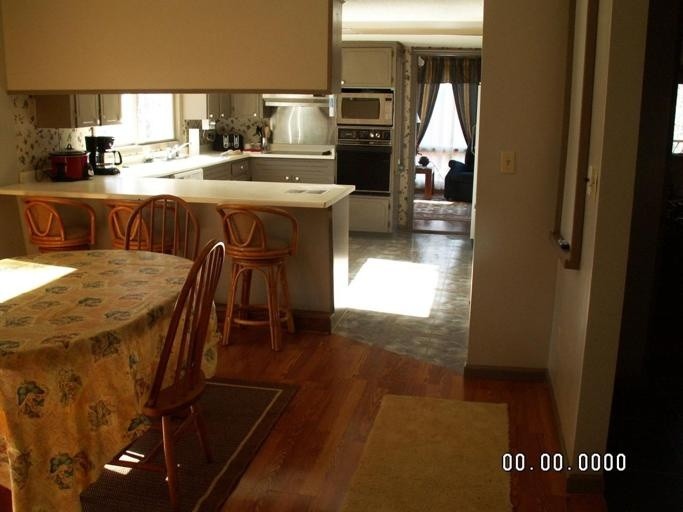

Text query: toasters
(212, 133), (243, 152)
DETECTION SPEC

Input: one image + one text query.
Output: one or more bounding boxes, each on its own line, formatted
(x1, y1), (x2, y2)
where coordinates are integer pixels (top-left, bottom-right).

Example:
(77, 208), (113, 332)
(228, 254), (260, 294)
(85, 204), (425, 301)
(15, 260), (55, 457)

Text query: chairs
(444, 148), (474, 202)
(105, 237), (225, 511)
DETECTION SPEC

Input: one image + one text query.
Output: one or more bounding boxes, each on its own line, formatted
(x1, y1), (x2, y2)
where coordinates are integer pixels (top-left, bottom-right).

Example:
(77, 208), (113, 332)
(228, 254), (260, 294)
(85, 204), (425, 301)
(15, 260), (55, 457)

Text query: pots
(45, 144), (89, 180)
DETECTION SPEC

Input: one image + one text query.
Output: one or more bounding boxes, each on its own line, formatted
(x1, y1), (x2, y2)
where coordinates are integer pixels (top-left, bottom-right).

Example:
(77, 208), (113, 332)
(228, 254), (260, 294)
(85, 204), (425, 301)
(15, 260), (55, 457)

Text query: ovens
(335, 143), (391, 196)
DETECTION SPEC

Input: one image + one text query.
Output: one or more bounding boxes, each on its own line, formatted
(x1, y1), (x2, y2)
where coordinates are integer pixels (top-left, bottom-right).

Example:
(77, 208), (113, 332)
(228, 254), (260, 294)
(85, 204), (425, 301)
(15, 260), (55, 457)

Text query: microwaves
(336, 92), (392, 126)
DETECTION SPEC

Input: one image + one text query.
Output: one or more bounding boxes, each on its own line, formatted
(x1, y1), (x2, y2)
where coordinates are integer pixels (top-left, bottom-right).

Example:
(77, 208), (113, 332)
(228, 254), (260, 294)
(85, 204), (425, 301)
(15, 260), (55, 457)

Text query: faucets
(165, 139), (193, 162)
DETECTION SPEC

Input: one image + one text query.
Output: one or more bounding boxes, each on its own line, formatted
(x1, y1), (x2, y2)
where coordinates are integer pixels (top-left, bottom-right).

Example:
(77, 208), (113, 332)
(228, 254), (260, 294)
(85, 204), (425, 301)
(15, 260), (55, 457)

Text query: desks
(0, 249), (215, 511)
(415, 168), (434, 199)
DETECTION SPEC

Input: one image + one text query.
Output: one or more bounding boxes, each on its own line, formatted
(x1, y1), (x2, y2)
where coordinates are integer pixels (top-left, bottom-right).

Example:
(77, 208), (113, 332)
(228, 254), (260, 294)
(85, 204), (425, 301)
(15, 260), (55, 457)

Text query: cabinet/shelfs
(33, 93), (121, 129)
(202, 158), (335, 185)
(339, 41), (405, 89)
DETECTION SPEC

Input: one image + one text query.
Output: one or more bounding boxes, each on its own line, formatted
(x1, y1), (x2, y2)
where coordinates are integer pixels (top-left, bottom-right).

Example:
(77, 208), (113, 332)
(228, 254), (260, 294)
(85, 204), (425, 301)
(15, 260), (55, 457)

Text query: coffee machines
(82, 135), (123, 175)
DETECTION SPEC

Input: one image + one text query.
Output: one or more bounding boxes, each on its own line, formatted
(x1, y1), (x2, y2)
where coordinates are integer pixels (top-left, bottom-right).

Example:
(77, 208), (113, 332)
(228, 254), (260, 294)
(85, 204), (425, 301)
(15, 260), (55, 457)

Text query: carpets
(80, 374), (301, 511)
(348, 256), (441, 318)
(340, 394), (513, 511)
